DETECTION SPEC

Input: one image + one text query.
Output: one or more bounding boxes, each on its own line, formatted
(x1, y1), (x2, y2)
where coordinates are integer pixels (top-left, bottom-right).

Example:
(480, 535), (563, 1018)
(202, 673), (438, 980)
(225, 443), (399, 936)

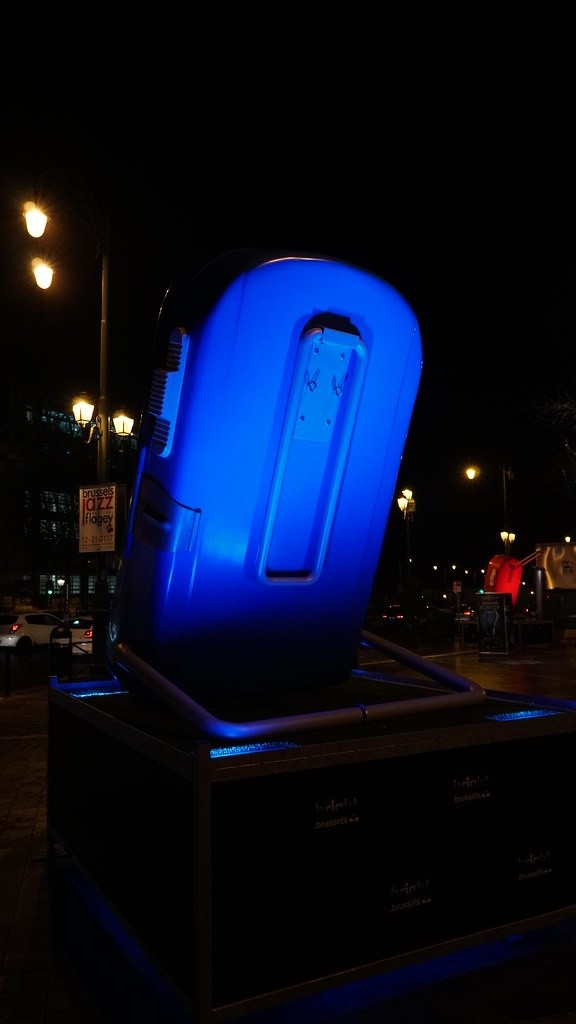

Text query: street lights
(17, 168), (134, 676)
(465, 462), (508, 533)
(397, 487), (415, 570)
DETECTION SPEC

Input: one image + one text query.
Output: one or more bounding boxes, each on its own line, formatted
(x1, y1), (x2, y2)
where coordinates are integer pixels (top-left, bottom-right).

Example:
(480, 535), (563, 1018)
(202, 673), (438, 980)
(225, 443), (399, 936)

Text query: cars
(61, 616), (93, 657)
(0, 612), (64, 657)
